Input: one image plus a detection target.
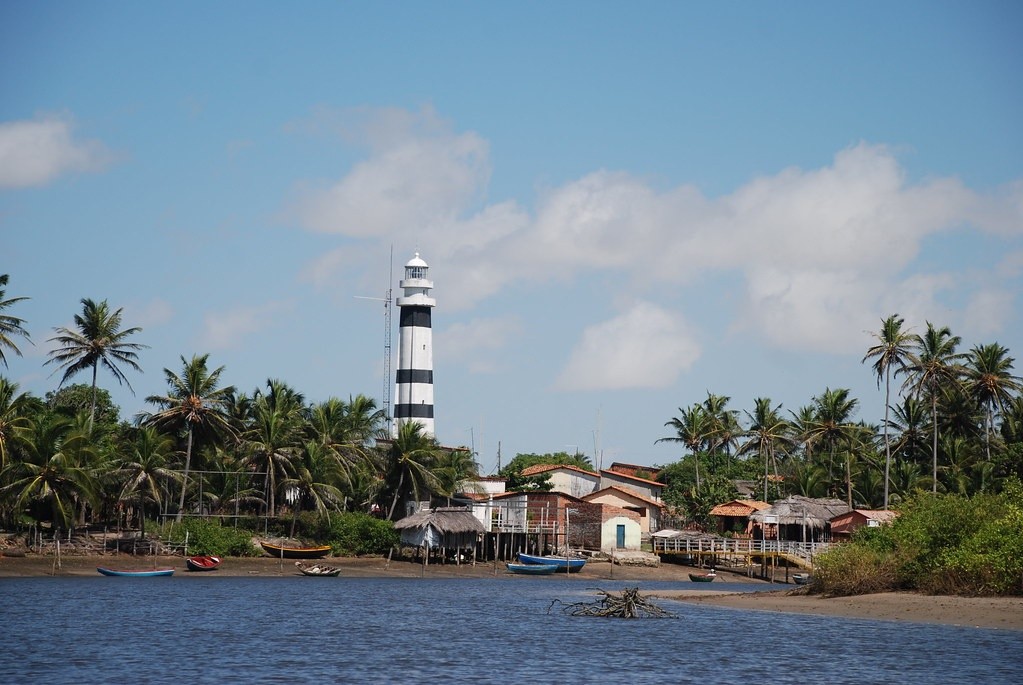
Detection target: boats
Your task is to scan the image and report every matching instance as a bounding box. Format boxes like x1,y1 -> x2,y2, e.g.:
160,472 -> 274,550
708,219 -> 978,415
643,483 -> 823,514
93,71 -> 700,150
504,561 -> 560,576
515,551 -> 587,573
793,573 -> 814,584
186,554 -> 222,571
295,561 -> 342,577
95,564 -> 177,577
687,572 -> 717,583
259,540 -> 334,559
